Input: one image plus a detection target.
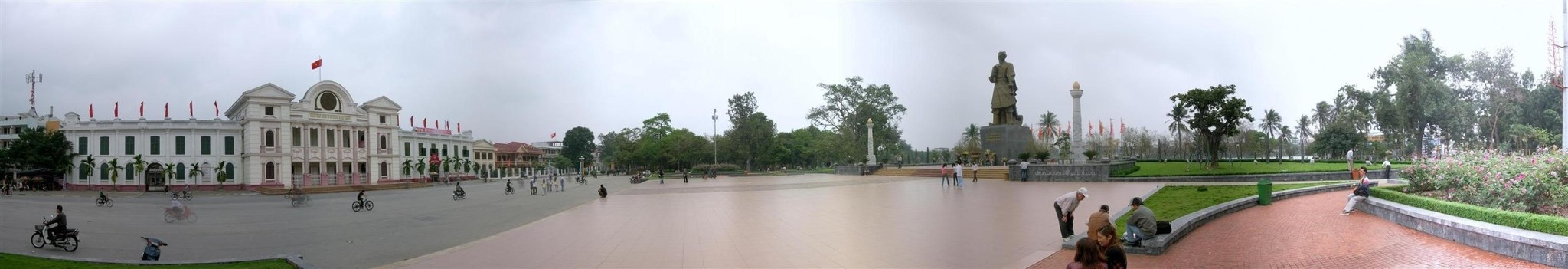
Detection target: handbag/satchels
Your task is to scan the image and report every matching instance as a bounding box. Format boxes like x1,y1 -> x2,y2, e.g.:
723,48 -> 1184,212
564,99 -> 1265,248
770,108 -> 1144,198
1155,220 -> 1172,234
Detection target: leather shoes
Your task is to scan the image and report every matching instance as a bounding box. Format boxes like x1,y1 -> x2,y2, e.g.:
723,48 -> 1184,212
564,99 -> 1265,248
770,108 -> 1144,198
1123,242 -> 1137,246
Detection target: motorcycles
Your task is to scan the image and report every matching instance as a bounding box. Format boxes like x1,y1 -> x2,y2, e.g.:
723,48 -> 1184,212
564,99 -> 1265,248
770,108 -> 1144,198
593,174 -> 598,178
290,195 -> 313,208
140,235 -> 167,261
533,176 -> 538,181
32,214 -> 81,252
629,170 -> 653,184
284,187 -> 303,199
164,204 -> 197,224
452,188 -> 467,201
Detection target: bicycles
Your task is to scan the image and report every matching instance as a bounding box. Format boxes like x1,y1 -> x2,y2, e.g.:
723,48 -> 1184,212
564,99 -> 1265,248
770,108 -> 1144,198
505,184 -> 515,195
580,178 -> 589,185
0,186 -> 13,197
171,191 -> 194,201
352,195 -> 374,212
96,194 -> 115,208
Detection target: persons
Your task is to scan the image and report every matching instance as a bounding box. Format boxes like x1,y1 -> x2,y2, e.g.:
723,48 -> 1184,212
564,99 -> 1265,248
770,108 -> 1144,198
3,174 -> 190,241
357,190 -> 368,209
1054,187 -> 1157,269
989,51 -> 1018,125
767,164 -> 801,172
290,184 -> 304,202
444,166 -> 716,198
898,154 -> 1031,190
1340,148 -> 1390,215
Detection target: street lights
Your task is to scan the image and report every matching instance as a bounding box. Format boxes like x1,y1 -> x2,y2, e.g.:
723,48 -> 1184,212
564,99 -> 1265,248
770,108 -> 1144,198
579,155 -> 585,177
1069,81 -> 1088,165
712,108 -> 719,166
631,159 -> 633,174
866,118 -> 875,165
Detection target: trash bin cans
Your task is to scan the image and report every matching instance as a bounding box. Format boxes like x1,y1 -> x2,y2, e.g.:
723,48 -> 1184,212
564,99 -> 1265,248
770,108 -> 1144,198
1352,168 -> 1359,180
1257,178 -> 1273,206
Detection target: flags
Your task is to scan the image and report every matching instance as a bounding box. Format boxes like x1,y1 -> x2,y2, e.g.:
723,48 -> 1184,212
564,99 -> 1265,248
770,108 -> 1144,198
1031,118 -> 1125,141
311,59 -> 322,69
89,101 -> 218,118
398,115 -> 460,132
551,132 -> 556,138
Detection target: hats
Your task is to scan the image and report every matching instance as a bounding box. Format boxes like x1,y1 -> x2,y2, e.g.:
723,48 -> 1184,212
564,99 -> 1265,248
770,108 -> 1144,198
1078,187 -> 1089,198
1130,197 -> 1142,206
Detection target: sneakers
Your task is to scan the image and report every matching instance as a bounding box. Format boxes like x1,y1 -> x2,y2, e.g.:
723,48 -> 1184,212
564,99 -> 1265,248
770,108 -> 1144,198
1069,234 -> 1075,238
1349,209 -> 1354,213
1339,209 -> 1349,215
1062,237 -> 1070,242
